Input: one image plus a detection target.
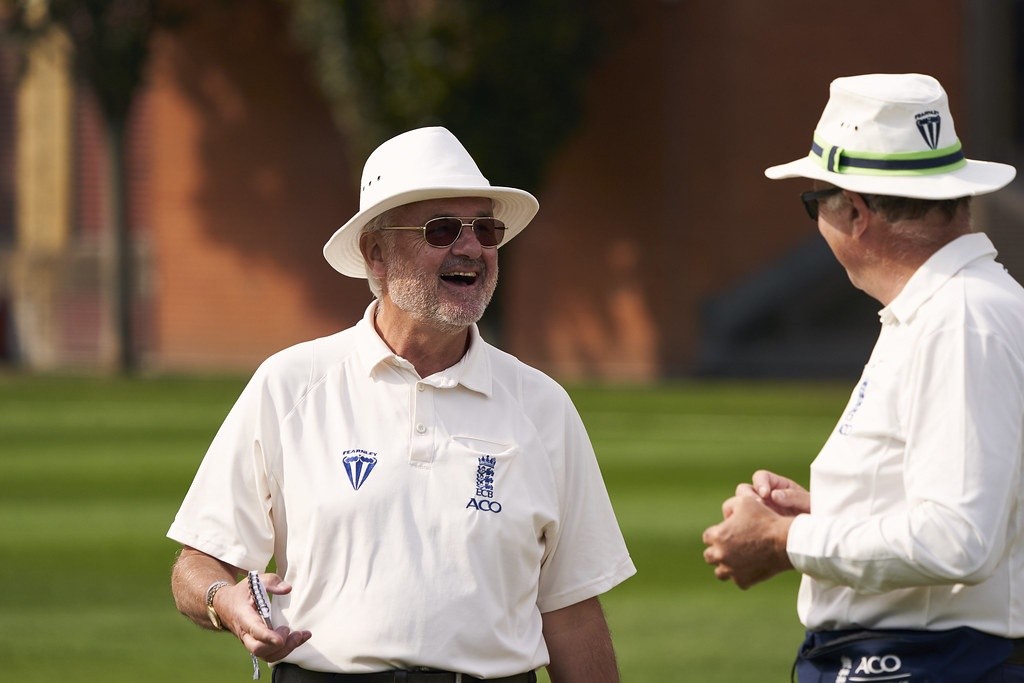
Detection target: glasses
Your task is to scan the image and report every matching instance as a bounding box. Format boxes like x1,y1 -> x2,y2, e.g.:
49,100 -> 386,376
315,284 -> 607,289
800,188 -> 842,221
379,217 -> 508,249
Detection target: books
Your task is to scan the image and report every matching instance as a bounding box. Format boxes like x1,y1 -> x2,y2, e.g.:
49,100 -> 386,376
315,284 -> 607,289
248,571 -> 274,630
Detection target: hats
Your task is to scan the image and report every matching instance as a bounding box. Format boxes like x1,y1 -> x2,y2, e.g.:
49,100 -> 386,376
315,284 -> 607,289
763,73 -> 1016,207
323,124 -> 541,280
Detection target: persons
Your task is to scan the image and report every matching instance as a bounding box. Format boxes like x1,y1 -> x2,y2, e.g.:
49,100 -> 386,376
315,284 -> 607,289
702,74 -> 1024,683
165,127 -> 638,683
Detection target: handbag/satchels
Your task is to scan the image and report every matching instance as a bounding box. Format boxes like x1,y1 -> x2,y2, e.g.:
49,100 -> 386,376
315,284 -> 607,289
790,622 -> 1017,683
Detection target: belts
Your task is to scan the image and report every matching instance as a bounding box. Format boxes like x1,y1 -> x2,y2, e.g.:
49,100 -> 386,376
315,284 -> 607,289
343,667 -> 537,683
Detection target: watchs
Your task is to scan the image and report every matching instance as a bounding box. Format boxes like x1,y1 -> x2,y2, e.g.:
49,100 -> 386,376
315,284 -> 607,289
206,581 -> 233,632
